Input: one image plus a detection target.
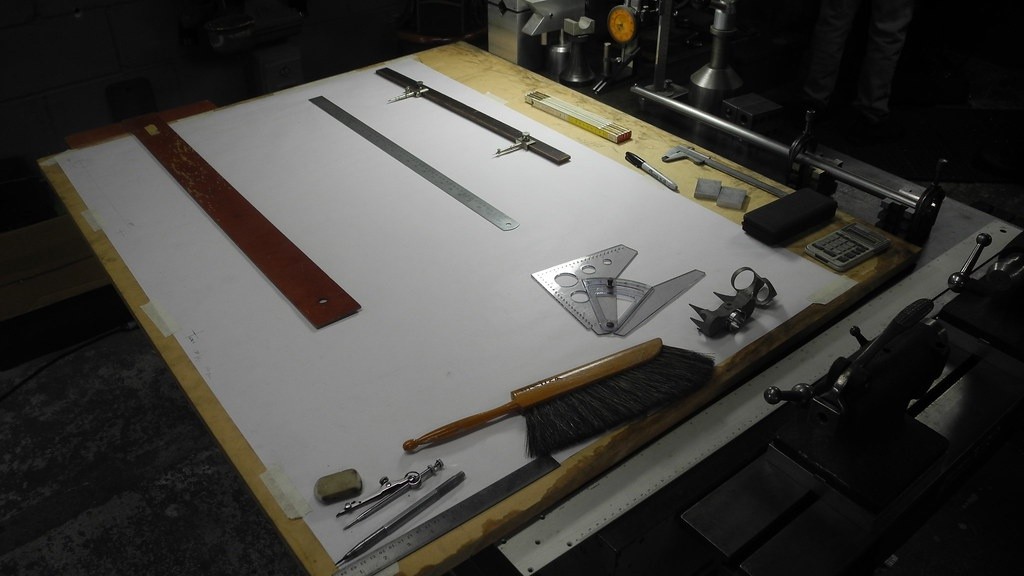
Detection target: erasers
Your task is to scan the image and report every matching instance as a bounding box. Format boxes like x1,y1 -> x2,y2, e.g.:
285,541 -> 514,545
313,469 -> 363,505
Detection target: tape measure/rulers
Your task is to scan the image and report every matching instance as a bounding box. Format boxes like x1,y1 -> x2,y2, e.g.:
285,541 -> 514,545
530,244 -> 707,336
308,96 -> 520,232
330,453 -> 561,576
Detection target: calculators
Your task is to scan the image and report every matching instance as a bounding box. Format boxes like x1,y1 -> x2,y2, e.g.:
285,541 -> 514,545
805,222 -> 892,272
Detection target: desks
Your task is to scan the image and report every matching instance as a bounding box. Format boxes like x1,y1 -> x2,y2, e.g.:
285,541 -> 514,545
35,34 -> 922,576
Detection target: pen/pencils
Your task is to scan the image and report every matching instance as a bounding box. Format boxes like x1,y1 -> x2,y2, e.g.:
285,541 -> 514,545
625,151 -> 678,191
